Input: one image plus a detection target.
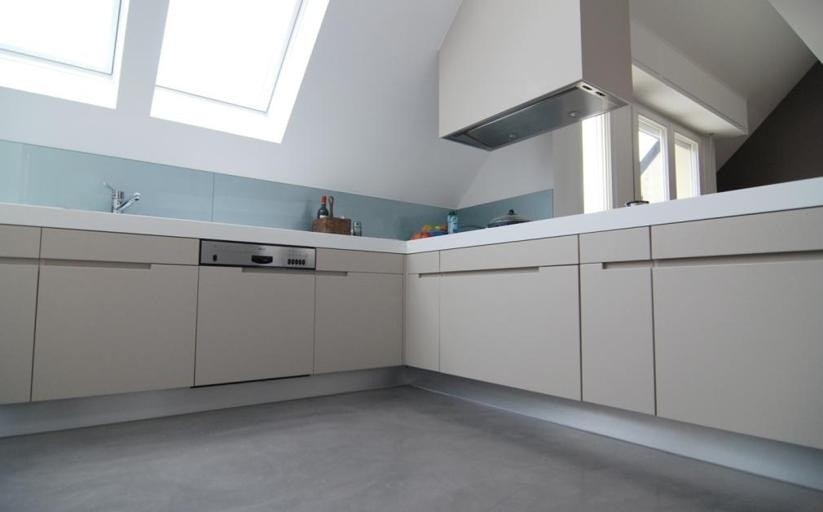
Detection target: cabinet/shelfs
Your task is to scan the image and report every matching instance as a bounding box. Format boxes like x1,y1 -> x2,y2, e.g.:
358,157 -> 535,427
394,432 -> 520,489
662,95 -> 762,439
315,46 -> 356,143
196,268 -> 314,390
651,205 -> 823,449
1,224 -> 200,408
402,249 -> 440,372
581,226 -> 656,416
315,248 -> 402,377
441,233 -> 580,402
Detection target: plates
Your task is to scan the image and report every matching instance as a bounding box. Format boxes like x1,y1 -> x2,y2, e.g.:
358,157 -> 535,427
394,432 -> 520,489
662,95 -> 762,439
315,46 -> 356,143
453,226 -> 481,231
426,231 -> 447,236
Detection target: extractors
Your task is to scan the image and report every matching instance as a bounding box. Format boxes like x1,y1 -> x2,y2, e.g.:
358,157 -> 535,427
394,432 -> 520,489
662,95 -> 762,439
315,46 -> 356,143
438,0 -> 633,152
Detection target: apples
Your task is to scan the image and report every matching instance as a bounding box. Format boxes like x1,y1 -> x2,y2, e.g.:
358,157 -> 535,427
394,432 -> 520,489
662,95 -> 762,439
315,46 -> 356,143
429,225 -> 447,231
410,233 -> 431,240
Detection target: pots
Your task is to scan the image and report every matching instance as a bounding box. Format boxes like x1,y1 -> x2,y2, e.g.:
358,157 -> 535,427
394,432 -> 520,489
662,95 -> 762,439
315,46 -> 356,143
488,208 -> 529,226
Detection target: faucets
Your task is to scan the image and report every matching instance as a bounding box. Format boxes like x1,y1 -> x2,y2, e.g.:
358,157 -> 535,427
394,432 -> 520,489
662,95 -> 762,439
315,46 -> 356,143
101,180 -> 141,212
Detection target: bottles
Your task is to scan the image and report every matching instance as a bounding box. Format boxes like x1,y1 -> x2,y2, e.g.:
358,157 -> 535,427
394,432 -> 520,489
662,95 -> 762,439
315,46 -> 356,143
317,192 -> 328,218
353,220 -> 362,236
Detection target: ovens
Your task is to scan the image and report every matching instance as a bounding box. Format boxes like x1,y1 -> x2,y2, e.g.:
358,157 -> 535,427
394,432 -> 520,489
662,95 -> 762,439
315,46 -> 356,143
197,237 -> 317,389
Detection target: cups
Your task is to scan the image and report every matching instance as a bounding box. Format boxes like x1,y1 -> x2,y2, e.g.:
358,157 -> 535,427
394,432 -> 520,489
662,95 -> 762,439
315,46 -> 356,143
446,209 -> 458,235
